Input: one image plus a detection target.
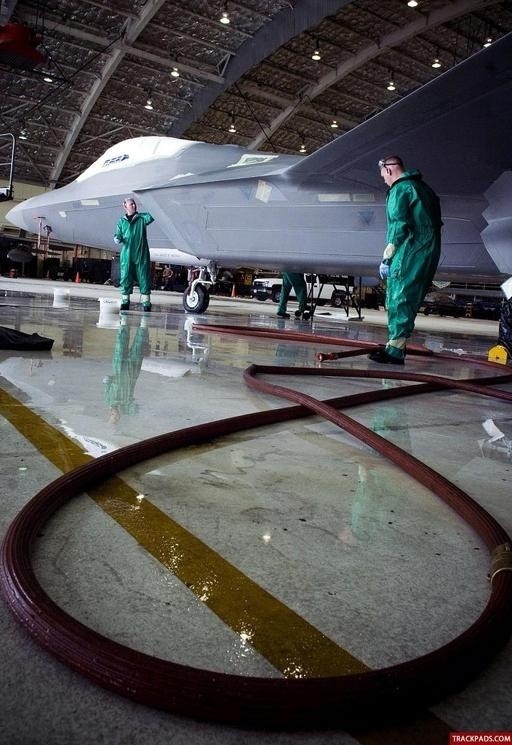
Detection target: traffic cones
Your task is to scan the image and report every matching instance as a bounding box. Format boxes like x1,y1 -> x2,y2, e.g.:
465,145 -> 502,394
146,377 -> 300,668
75,272 -> 81,284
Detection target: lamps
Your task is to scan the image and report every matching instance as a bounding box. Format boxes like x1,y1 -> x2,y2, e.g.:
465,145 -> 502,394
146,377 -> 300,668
387,0 -> 493,92
44,71 -> 53,84
18,130 -> 28,140
144,100 -> 154,110
220,0 -> 230,24
311,40 -> 321,61
299,138 -> 307,153
170,68 -> 180,78
229,116 -> 237,133
330,110 -> 339,129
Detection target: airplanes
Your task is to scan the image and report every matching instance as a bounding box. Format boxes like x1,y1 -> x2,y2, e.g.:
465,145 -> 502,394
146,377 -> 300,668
4,32 -> 511,313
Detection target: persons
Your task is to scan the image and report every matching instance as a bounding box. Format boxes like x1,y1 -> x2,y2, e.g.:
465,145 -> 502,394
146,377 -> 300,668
277,271 -> 315,318
161,264 -> 174,291
271,317 -> 310,368
221,268 -> 234,293
366,154 -> 444,365
334,364 -> 412,557
113,198 -> 157,311
101,310 -> 152,427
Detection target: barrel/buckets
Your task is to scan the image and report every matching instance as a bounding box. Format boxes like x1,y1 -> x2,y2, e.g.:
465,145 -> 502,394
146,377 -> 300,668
54,288 -> 71,309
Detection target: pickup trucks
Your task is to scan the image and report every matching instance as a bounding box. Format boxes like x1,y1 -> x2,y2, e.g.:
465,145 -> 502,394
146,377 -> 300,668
249,272 -> 357,308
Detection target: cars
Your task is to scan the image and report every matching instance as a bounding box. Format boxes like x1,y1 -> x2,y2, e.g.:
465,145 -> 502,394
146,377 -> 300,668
419,291 -> 468,318
471,300 -> 502,321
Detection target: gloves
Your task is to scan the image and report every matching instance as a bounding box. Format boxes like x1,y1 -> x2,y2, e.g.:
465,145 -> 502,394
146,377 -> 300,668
114,236 -> 121,246
379,262 -> 390,280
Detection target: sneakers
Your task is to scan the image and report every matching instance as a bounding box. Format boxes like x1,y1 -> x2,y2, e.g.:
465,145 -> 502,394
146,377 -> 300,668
370,350 -> 404,365
144,306 -> 153,313
277,312 -> 290,317
121,304 -> 129,310
